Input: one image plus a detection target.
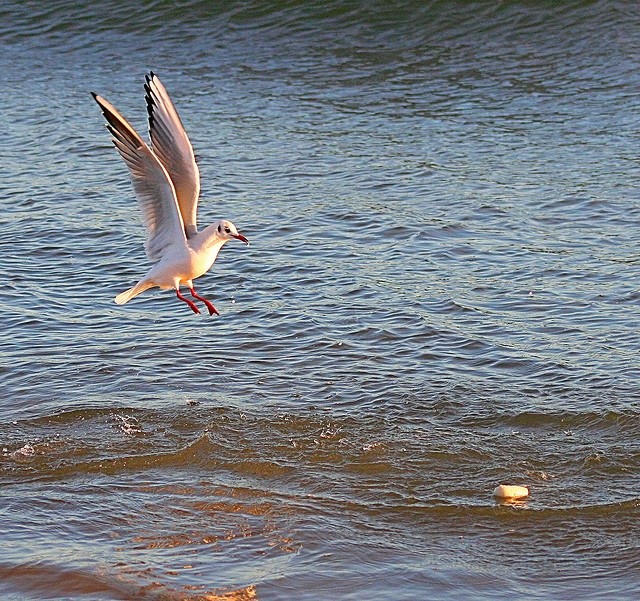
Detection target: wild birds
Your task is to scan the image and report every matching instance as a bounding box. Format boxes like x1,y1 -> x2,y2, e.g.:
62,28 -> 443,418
86,68 -> 252,319
